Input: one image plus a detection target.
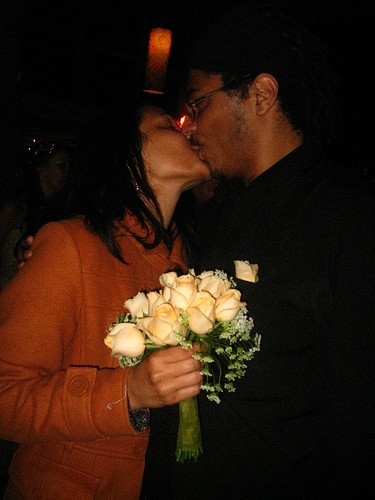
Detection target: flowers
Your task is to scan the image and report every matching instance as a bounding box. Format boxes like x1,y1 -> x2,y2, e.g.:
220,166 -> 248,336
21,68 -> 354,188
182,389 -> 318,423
105,259 -> 262,463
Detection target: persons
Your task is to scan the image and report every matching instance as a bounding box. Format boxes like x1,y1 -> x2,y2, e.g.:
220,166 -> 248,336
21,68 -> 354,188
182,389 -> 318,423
14,8 -> 375,500
0,103 -> 211,500
0,141 -> 75,288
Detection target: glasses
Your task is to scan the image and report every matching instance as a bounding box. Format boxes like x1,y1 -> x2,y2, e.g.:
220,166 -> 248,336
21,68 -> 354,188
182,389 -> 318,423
173,73 -> 258,128
